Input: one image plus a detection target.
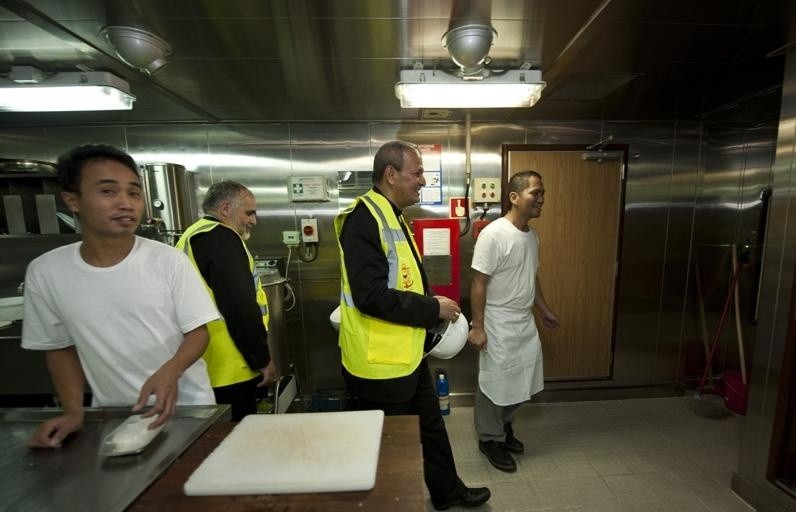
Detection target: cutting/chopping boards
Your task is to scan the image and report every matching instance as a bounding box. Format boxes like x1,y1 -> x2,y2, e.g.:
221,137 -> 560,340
180,410 -> 385,495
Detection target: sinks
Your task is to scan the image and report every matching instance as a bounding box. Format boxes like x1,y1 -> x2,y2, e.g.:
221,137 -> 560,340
0,297 -> 25,328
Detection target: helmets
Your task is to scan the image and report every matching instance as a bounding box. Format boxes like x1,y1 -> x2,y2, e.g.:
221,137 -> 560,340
421,312 -> 470,362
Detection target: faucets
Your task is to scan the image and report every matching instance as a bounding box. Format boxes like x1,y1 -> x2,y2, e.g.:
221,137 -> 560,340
17,281 -> 26,294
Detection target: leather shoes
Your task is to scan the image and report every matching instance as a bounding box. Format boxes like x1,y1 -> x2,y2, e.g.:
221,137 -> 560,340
479,438 -> 517,473
503,423 -> 525,452
434,488 -> 490,512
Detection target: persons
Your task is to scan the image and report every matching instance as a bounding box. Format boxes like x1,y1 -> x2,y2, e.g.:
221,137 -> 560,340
20,144 -> 220,449
174,181 -> 277,422
339,141 -> 490,510
468,171 -> 559,471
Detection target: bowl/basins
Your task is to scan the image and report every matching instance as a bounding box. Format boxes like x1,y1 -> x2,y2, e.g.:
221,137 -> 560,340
1,297 -> 21,324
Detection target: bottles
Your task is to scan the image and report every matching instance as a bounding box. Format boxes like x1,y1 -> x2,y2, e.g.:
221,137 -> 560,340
435,369 -> 451,416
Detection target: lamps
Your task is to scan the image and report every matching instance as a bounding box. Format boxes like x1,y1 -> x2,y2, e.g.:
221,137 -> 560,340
394,68 -> 547,110
1,70 -> 138,114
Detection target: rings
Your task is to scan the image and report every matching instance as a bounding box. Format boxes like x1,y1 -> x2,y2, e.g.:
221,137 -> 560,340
454,311 -> 460,318
